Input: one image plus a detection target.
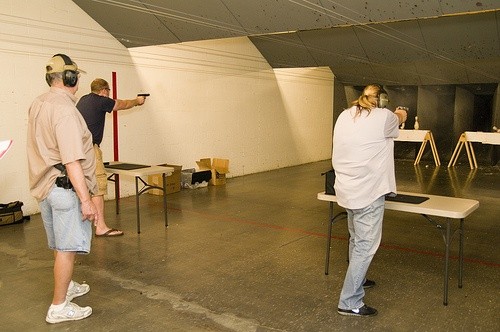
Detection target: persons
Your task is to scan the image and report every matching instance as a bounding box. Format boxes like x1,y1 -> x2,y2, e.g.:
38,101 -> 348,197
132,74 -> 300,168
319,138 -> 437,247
75,78 -> 146,236
26,54 -> 100,324
332,85 -> 407,317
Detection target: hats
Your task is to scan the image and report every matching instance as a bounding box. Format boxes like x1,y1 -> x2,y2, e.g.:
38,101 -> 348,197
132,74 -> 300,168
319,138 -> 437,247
45,53 -> 87,73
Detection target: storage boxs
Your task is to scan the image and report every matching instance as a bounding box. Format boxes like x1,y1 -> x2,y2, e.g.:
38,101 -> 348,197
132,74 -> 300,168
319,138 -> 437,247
147,164 -> 182,195
180,168 -> 211,190
196,157 -> 230,186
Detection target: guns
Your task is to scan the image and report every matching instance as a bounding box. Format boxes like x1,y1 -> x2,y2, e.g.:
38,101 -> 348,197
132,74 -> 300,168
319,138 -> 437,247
138,94 -> 150,96
397,106 -> 409,111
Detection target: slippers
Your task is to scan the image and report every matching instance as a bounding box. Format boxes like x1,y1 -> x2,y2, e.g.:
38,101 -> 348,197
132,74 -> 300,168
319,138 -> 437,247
95,228 -> 124,237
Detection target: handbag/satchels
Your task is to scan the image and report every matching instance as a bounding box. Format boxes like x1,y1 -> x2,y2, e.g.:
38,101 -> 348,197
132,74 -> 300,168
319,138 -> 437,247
0,200 -> 30,226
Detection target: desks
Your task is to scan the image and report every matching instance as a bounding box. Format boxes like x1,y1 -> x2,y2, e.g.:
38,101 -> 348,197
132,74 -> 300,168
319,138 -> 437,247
447,131 -> 500,170
317,191 -> 480,306
392,129 -> 440,167
100,161 -> 175,234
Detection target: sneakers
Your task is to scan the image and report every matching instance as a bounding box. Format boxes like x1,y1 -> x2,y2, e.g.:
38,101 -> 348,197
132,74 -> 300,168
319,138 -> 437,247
338,304 -> 378,317
362,279 -> 376,288
45,300 -> 93,324
66,280 -> 91,301
